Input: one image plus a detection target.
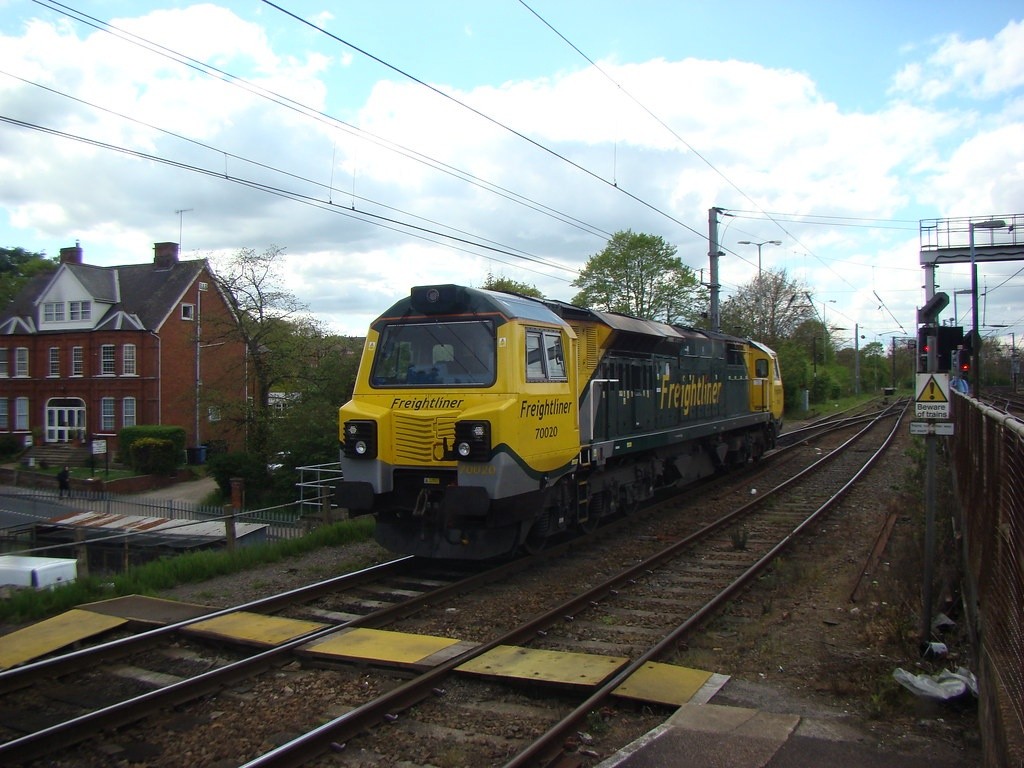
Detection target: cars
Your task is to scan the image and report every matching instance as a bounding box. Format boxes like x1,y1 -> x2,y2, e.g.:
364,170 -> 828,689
266,451 -> 292,477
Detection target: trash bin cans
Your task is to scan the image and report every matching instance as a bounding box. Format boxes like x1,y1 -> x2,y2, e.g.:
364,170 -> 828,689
193,446 -> 208,465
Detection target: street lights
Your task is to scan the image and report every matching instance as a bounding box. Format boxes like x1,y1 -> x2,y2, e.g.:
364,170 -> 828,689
736,238 -> 783,343
968,220 -> 1006,400
822,299 -> 837,365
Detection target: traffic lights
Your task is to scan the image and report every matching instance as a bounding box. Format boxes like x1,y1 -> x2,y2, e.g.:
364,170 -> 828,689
958,350 -> 970,373
917,326 -> 938,373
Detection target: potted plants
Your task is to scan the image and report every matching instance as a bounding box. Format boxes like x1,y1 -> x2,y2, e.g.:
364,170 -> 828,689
65,422 -> 81,447
33,425 -> 44,446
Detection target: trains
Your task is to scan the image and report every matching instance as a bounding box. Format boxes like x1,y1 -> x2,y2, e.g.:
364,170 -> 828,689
336,283 -> 784,566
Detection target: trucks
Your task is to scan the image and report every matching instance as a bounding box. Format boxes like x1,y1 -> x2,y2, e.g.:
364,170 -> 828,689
0,554 -> 78,590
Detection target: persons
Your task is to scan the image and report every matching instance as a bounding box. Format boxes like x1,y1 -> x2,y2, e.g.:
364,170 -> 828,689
57,465 -> 71,499
949,375 -> 968,396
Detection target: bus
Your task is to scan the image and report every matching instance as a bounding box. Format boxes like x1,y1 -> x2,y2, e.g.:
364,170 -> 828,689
267,393 -> 303,407
